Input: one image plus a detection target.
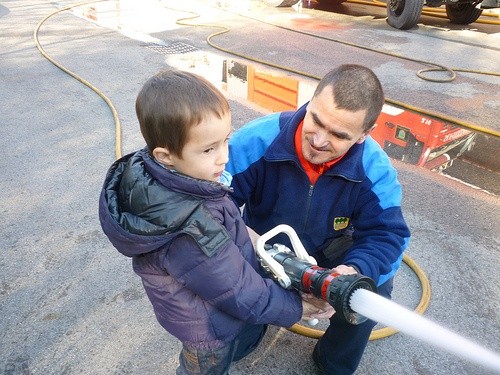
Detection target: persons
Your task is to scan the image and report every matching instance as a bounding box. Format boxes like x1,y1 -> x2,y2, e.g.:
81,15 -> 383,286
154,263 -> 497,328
214,63 -> 412,375
97,69 -> 328,375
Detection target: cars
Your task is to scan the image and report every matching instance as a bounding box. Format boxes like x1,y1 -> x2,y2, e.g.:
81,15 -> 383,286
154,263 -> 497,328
271,0 -> 500,31
